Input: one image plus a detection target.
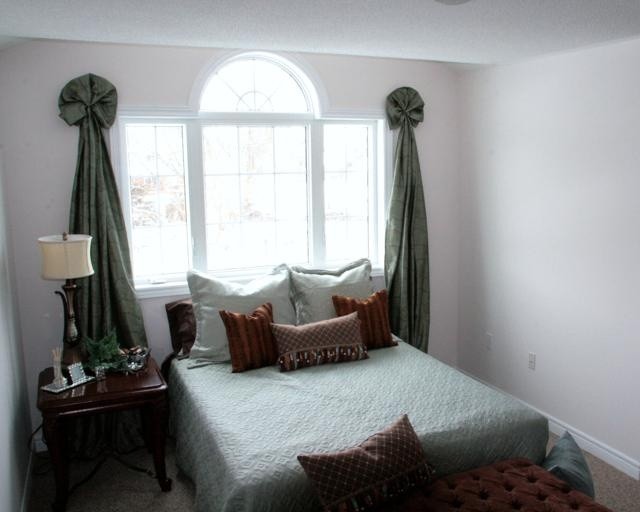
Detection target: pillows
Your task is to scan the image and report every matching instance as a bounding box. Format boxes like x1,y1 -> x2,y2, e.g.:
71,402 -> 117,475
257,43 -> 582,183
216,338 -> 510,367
297,415 -> 439,512
166,259 -> 399,372
542,430 -> 594,500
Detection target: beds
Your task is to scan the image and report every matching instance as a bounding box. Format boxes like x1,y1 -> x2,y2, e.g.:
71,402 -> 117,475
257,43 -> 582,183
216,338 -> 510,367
168,342 -> 549,512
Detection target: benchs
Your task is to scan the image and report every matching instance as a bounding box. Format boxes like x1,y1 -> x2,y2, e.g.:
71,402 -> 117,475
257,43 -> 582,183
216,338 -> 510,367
395,454 -> 612,512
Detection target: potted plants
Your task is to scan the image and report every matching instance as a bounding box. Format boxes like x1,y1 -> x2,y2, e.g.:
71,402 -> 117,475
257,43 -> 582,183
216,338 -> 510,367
82,328 -> 128,372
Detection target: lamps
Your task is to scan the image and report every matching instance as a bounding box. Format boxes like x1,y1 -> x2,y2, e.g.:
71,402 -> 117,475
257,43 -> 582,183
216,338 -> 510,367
38,233 -> 97,369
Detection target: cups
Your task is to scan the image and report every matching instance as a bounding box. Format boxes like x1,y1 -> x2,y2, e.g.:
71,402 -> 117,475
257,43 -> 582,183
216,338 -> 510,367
127,347 -> 152,375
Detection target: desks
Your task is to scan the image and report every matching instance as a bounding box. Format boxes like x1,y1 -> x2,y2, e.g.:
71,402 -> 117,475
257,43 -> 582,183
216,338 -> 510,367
37,356 -> 172,512
